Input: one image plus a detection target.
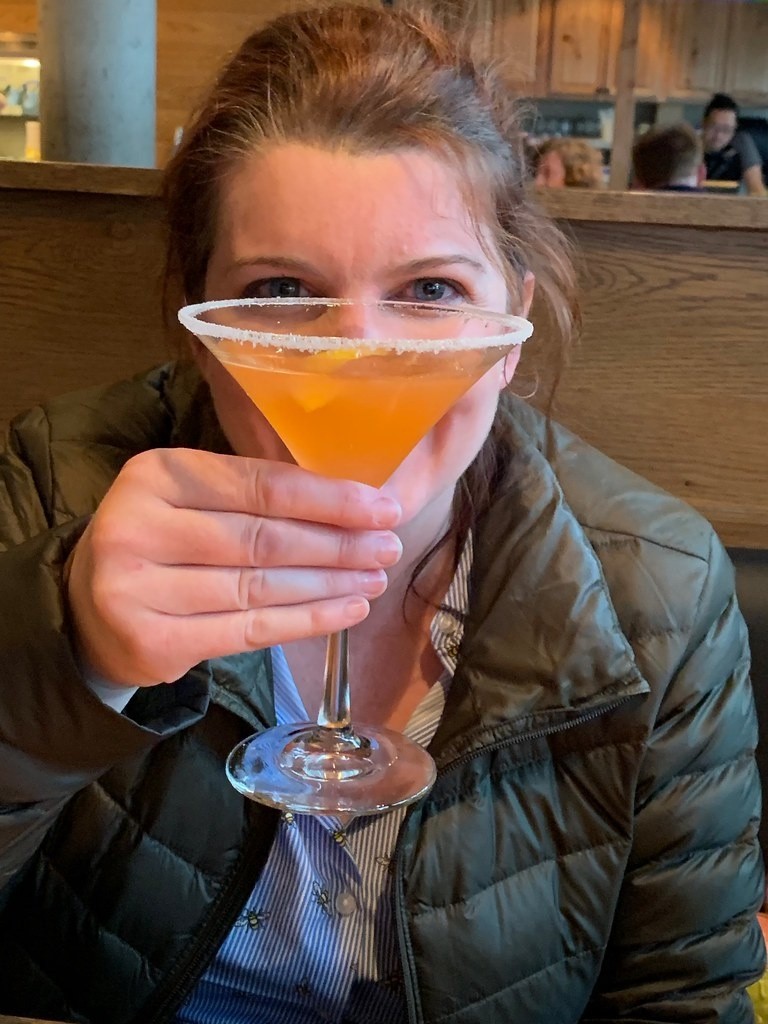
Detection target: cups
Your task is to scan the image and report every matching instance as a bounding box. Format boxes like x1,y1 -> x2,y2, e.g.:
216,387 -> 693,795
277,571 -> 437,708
0,28 -> 44,158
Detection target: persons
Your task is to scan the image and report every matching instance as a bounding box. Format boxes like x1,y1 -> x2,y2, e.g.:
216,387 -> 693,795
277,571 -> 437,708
692,93 -> 767,198
633,121 -> 713,194
530,137 -> 607,191
1,1 -> 768,1023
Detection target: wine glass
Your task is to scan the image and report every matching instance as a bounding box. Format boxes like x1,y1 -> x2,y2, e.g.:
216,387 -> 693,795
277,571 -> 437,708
170,294 -> 534,816
